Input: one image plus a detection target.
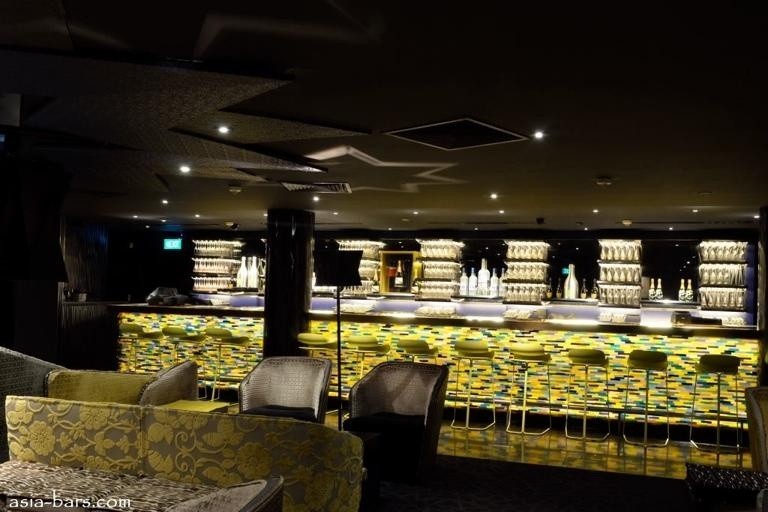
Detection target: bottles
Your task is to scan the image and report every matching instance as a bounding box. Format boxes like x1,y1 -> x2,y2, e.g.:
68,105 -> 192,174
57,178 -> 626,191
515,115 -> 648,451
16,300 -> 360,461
656,278 -> 663,300
686,279 -> 694,302
649,278 -> 655,303
556,277 -> 563,299
563,264 -> 579,300
677,277 -> 686,302
394,259 -> 403,288
590,278 -> 598,300
339,285 -> 367,299
579,278 -> 587,298
459,258 -> 504,299
236,255 -> 264,291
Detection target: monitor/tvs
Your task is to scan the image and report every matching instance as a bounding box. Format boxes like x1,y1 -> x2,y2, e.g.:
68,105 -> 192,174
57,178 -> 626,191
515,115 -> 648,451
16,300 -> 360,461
162,237 -> 182,252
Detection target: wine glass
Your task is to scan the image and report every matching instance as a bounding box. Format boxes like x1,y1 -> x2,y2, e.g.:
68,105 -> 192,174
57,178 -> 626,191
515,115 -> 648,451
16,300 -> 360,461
695,241 -> 748,312
503,239 -> 550,304
191,239 -> 241,292
416,237 -> 462,302
598,239 -> 643,308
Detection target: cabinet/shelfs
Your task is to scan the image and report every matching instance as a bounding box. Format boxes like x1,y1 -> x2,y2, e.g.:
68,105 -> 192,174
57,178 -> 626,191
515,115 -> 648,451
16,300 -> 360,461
502,238 -> 550,304
597,236 -> 642,308
187,234 -> 241,293
339,234 -> 464,301
697,240 -> 749,311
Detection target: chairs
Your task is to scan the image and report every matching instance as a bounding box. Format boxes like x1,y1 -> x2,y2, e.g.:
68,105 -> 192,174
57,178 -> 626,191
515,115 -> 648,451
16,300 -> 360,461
0,346 -> 451,510
115,318 -> 256,401
296,330 -> 746,475
